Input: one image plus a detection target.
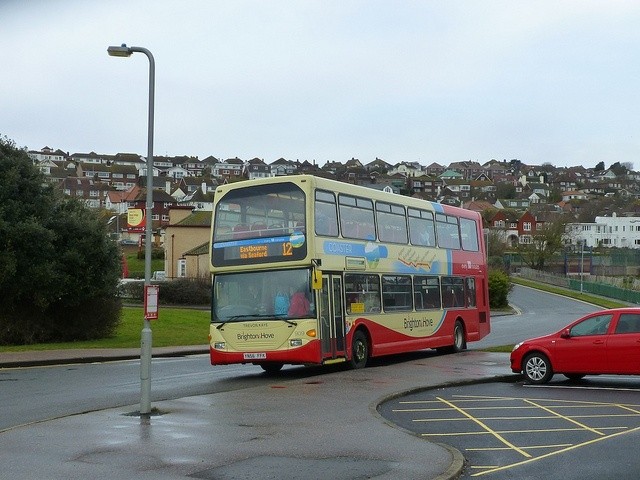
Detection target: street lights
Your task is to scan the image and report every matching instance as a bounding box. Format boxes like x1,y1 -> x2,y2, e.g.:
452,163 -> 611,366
106,43 -> 155,413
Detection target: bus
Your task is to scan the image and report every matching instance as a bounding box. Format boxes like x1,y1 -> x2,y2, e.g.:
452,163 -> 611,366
208,174 -> 491,369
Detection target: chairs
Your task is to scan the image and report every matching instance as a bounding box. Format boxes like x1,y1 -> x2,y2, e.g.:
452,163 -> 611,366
268,224 -> 283,236
250,221 -> 267,238
232,223 -> 250,238
295,220 -> 405,243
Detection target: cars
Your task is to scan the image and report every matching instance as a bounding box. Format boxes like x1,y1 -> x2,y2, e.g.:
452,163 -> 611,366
510,307 -> 640,385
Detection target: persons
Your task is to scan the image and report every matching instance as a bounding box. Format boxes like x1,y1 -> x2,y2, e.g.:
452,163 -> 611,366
420,228 -> 431,247
245,283 -> 259,305
275,285 -> 289,315
289,285 -> 310,317
